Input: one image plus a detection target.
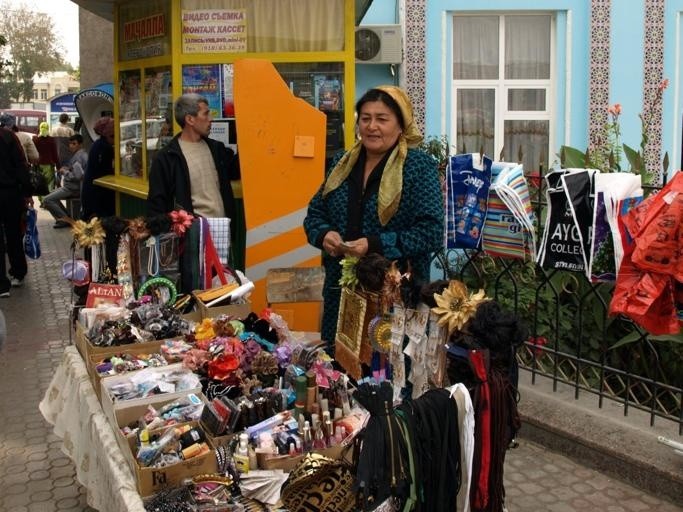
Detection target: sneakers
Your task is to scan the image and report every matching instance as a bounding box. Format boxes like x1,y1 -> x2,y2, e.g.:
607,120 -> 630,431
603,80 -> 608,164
0,271 -> 23,298
53,220 -> 72,228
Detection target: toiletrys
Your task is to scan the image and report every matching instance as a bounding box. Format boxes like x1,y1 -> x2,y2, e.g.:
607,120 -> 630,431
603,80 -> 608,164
234,373 -> 346,470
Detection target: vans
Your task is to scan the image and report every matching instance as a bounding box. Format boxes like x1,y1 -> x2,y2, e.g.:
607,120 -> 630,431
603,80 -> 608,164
0,108 -> 47,135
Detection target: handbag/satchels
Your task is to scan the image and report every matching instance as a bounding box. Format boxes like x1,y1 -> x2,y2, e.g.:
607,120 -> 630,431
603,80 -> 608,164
24,208 -> 42,260
444,152 -> 643,286
280,452 -> 359,512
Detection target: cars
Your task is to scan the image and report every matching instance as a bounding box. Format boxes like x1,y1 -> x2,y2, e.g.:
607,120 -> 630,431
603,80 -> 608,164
119,118 -> 161,175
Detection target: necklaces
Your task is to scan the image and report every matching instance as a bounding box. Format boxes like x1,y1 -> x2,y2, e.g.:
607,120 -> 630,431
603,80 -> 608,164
145,230 -> 177,278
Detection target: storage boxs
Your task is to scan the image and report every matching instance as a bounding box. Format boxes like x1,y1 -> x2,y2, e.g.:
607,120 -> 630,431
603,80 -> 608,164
200,421 -> 352,473
180,289 -> 250,322
75,319 -> 218,498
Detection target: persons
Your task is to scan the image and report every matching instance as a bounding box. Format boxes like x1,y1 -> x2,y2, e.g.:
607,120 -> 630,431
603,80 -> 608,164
36,121 -> 56,207
0,124 -> 34,297
43,134 -> 89,228
303,86 -> 445,379
52,114 -> 74,188
81,114 -> 114,262
12,124 -> 40,209
0,112 -> 16,253
145,93 -> 241,273
